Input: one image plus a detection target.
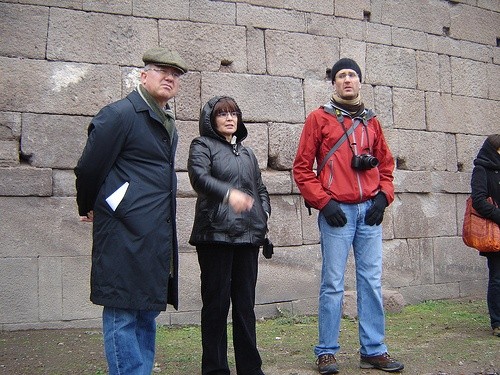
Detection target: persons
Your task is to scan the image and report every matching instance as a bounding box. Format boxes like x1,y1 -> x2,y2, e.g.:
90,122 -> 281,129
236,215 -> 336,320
74,48 -> 189,375
187,96 -> 271,375
471,134 -> 500,335
293,58 -> 404,374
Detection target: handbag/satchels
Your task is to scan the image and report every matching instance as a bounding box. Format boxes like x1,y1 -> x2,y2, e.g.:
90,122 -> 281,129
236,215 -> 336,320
462,194 -> 500,252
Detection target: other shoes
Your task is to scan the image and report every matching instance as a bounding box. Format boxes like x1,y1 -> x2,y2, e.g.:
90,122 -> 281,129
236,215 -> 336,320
494,325 -> 500,337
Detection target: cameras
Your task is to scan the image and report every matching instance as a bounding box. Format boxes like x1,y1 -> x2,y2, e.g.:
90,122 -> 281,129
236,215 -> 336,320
351,153 -> 379,170
263,237 -> 273,259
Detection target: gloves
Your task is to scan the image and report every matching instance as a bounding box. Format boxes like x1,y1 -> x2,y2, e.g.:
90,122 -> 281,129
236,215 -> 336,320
365,193 -> 388,226
321,199 -> 348,227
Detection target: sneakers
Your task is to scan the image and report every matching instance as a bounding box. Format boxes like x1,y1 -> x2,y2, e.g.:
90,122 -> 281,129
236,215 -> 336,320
360,352 -> 404,372
315,353 -> 339,374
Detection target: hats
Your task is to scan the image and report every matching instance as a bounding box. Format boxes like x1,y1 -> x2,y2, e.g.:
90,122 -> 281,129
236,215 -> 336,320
142,47 -> 188,74
332,58 -> 362,84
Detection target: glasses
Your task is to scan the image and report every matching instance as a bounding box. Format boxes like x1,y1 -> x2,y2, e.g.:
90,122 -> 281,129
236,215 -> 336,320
217,112 -> 237,119
147,68 -> 181,80
